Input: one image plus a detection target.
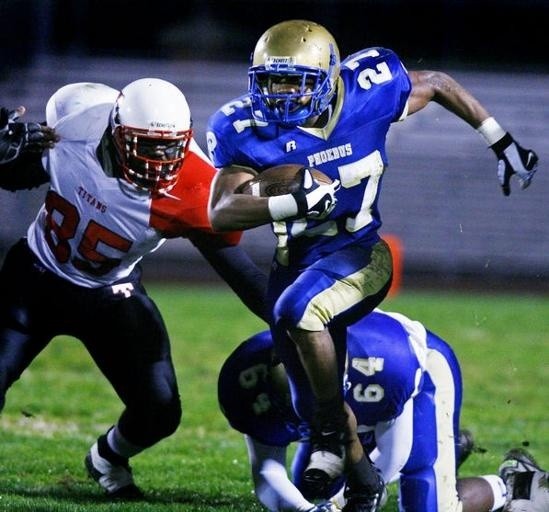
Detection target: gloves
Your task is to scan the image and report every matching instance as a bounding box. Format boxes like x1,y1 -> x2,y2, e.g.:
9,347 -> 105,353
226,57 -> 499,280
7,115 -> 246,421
307,502 -> 340,512
480,115 -> 537,196
0,107 -> 59,163
269,169 -> 342,221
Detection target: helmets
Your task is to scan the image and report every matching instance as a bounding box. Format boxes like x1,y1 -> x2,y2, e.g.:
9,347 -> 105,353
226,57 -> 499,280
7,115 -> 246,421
262,362 -> 304,425
110,78 -> 193,193
248,20 -> 341,123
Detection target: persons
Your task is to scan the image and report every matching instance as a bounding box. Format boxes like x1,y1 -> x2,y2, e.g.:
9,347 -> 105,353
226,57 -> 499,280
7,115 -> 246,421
2,77 -> 272,499
205,18 -> 539,512
219,305 -> 546,511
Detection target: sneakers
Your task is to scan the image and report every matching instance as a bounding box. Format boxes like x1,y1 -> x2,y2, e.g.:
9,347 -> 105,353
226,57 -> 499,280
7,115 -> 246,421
500,448 -> 542,478
339,475 -> 387,512
84,434 -> 141,500
302,430 -> 345,498
455,431 -> 472,470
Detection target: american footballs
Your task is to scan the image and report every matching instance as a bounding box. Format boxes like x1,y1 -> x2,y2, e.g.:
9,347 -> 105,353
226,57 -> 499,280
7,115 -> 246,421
240,164 -> 333,197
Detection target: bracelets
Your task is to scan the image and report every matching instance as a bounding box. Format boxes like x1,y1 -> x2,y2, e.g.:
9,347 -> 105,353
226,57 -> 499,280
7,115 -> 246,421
264,194 -> 299,220
476,115 -> 505,145
